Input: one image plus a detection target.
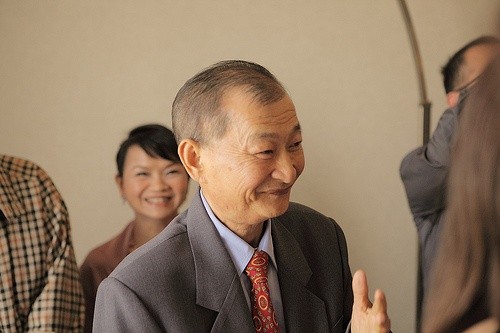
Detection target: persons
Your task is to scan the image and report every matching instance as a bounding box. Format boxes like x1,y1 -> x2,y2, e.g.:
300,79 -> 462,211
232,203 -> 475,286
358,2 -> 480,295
0,153 -> 88,333
421,51 -> 500,332
399,35 -> 500,308
91,59 -> 394,333
79,123 -> 189,333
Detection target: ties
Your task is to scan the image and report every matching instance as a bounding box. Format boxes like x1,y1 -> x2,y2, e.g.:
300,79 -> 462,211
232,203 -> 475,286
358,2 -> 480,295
244,250 -> 282,333
